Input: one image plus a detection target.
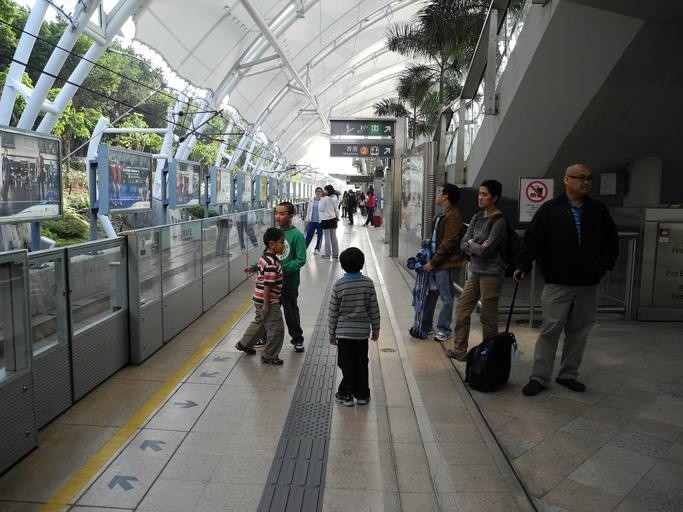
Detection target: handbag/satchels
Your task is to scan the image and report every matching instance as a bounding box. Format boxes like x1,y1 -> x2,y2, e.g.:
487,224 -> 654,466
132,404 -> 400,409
464,332 -> 514,392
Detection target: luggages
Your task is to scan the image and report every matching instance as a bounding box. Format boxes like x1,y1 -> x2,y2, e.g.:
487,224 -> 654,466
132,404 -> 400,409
373,210 -> 381,227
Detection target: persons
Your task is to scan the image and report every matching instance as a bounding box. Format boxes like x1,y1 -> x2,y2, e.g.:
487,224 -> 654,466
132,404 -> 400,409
235,200 -> 258,250
215,212 -> 233,257
242,201 -> 306,352
421,183 -> 466,340
235,227 -> 284,366
304,185 -> 379,258
328,247 -> 380,406
513,164 -> 619,396
0,148 -> 57,201
138,176 -> 151,202
446,179 -> 508,362
109,158 -> 128,206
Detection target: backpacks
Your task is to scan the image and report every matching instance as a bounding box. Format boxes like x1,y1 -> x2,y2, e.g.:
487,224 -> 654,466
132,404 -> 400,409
487,214 -> 519,265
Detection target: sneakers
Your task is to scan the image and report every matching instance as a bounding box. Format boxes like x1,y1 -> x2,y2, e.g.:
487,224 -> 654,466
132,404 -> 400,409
234,342 -> 255,355
555,378 -> 585,392
332,255 -> 337,258
260,355 -> 283,365
253,338 -> 265,348
522,379 -> 543,396
320,255 -> 329,259
294,343 -> 304,352
445,349 -> 466,362
357,396 -> 369,405
433,331 -> 447,341
313,249 -> 318,255
334,392 -> 353,407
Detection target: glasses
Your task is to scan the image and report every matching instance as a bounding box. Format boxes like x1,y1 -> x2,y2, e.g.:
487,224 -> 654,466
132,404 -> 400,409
566,174 -> 592,180
